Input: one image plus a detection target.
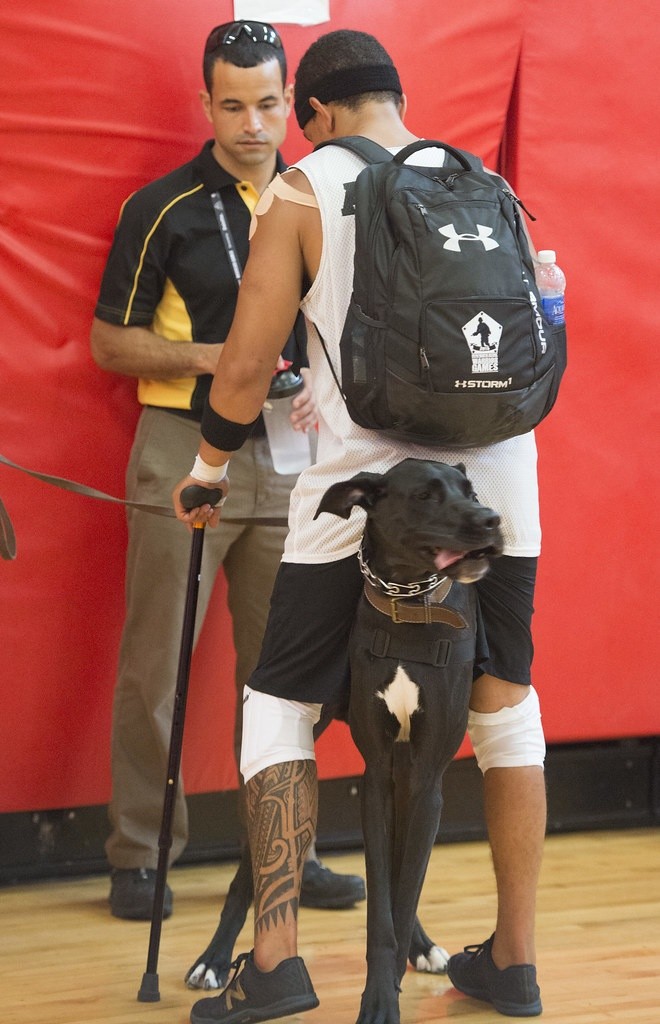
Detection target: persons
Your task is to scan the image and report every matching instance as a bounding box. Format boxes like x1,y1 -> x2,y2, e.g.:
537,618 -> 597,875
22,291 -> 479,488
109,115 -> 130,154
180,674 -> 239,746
88,23 -> 367,920
172,32 -> 546,1024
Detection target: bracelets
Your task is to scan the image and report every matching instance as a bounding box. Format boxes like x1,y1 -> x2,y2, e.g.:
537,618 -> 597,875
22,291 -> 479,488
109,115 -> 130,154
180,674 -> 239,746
187,454 -> 230,485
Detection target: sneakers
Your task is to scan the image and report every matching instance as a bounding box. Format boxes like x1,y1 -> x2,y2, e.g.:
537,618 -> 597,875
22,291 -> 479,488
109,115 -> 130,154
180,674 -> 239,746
447,932 -> 542,1016
108,867 -> 172,919
298,856 -> 366,907
190,947 -> 320,1024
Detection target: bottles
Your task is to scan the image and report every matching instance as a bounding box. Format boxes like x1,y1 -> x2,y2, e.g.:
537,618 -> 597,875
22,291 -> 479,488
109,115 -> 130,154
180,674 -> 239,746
534,249 -> 566,325
262,359 -> 312,473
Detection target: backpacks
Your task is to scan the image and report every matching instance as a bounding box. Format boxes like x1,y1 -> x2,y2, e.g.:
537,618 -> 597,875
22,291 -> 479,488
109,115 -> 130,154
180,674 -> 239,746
313,136 -> 567,450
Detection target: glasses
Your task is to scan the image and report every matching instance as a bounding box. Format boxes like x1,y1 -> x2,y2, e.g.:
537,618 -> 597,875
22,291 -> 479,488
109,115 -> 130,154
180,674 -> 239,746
205,21 -> 283,53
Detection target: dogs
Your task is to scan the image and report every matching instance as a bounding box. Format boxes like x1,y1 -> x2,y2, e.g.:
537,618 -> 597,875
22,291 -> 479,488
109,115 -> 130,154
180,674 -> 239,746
182,458 -> 504,1024
